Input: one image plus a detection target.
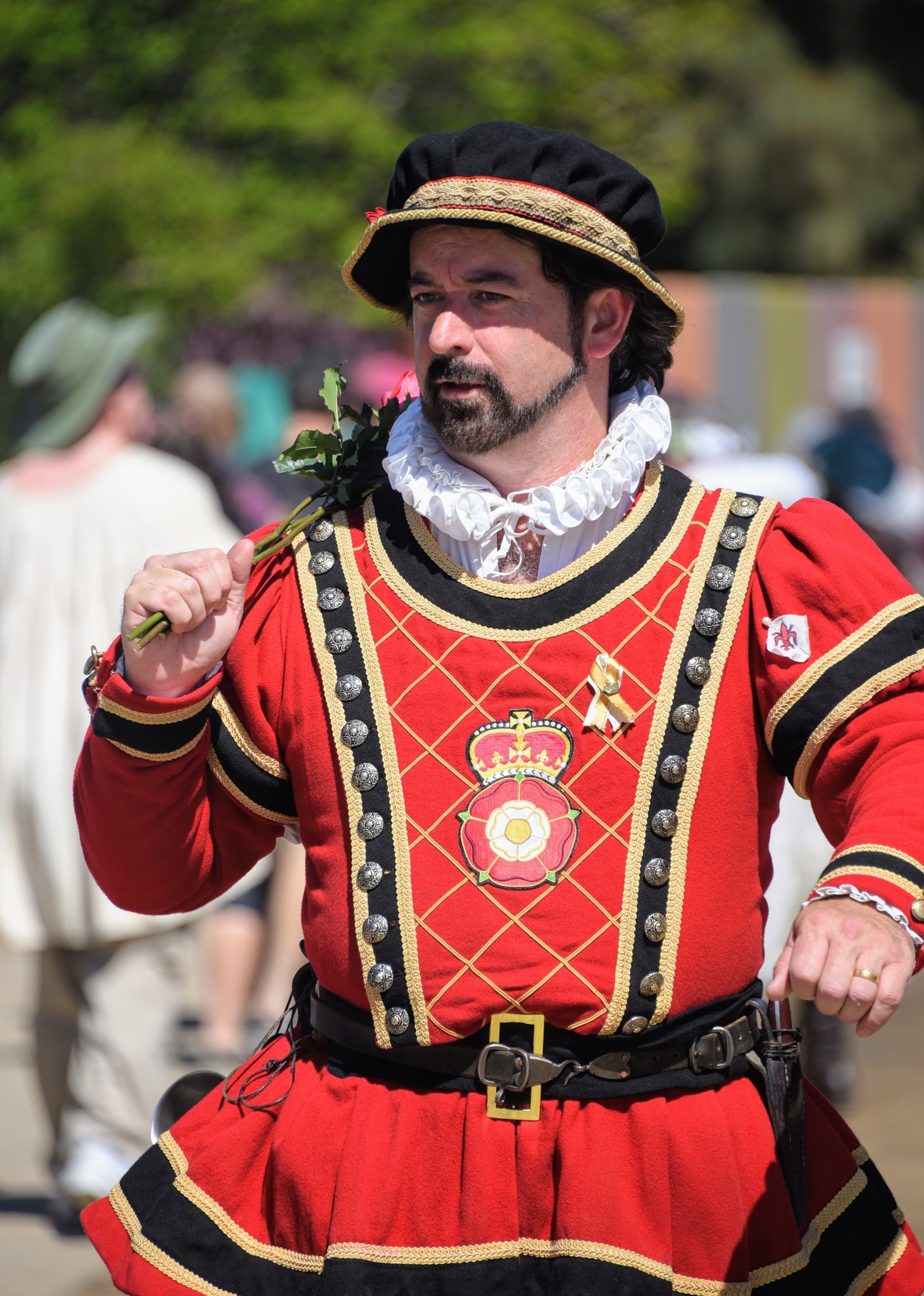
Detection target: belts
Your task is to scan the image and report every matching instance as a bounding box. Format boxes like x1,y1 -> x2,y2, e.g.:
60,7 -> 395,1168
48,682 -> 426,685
308,993 -> 760,1101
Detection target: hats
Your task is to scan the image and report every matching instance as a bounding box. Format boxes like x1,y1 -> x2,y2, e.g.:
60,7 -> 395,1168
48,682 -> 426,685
341,120 -> 684,345
8,295 -> 161,453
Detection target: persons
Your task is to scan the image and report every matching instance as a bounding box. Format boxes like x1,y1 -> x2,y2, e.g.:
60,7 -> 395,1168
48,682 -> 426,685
0,120 -> 924,1296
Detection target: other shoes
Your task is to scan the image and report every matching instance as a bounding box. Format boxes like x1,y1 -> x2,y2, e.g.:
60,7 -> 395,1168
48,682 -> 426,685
46,1193 -> 90,1236
0,1198 -> 46,1212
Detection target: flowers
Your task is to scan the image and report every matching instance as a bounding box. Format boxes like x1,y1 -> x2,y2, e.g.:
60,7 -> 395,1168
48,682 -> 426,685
125,360 -> 418,650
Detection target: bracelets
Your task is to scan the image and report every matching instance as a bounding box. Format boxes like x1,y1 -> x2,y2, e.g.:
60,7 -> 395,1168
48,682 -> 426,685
853,968 -> 879,985
